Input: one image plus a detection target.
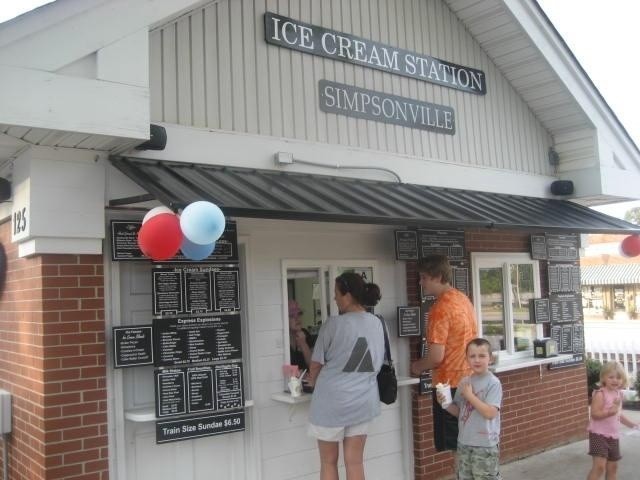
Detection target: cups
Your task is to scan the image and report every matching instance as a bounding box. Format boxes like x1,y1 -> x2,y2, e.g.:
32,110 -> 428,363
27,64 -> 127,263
288,380 -> 303,397
436,384 -> 453,404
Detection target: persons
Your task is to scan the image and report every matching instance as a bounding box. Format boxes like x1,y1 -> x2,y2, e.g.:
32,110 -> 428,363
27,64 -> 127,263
436,338 -> 504,480
302,272 -> 390,480
586,359 -> 640,480
411,254 -> 479,480
288,299 -> 318,373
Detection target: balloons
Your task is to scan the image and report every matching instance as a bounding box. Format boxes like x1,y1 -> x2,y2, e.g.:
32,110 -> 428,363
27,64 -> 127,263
180,201 -> 225,245
179,233 -> 215,261
142,205 -> 181,226
138,213 -> 183,260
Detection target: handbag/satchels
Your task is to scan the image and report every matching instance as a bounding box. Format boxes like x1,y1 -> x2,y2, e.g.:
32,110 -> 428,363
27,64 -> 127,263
377,364 -> 397,404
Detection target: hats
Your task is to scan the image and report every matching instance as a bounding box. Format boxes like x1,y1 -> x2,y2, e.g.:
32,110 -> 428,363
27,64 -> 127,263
288,300 -> 302,317
